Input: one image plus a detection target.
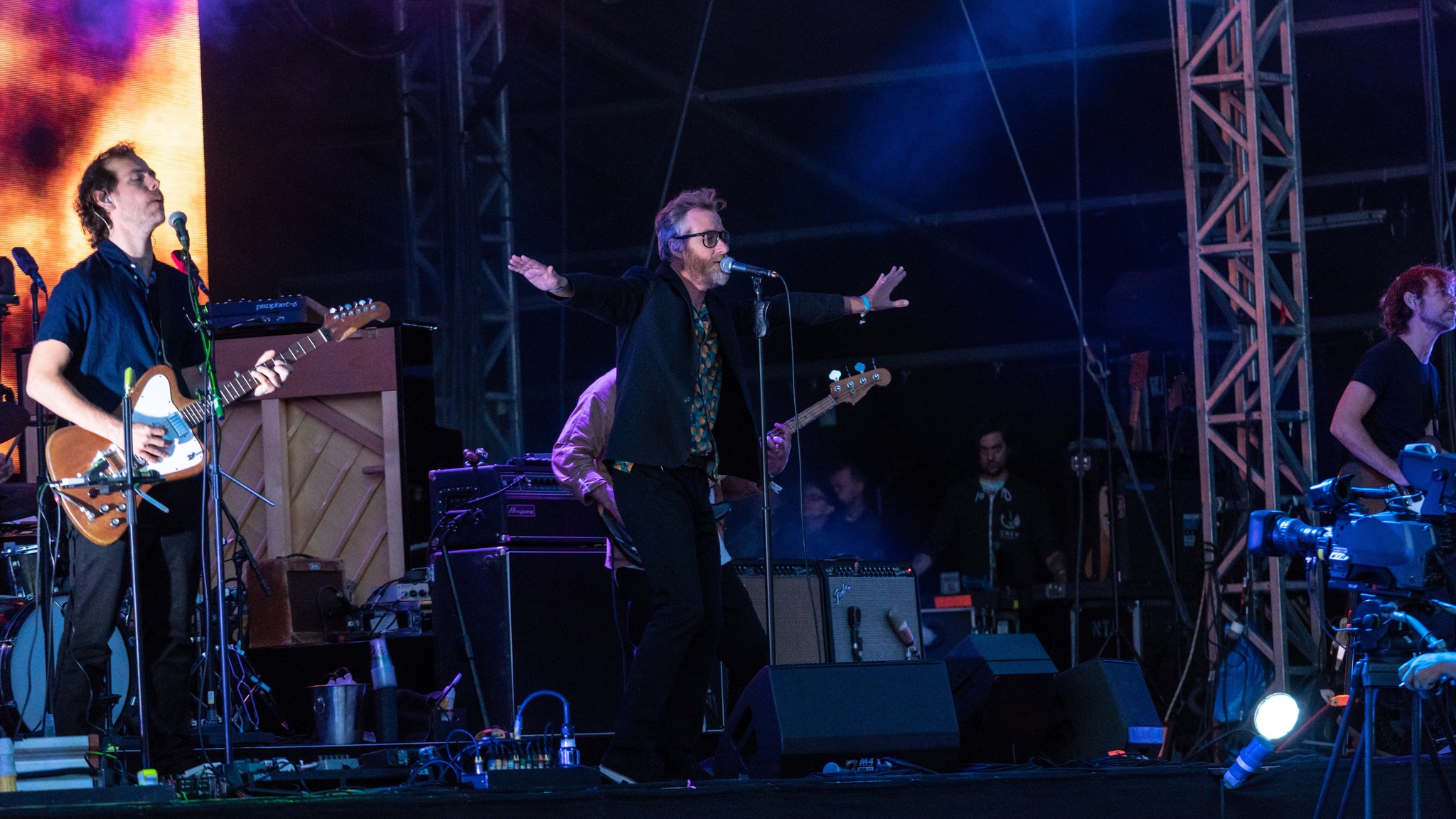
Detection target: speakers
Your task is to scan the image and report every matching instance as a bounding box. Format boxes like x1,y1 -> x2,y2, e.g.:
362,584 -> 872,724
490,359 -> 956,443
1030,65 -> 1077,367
941,632 -> 1061,763
1051,658 -> 1166,762
888,606 -> 922,657
243,552 -> 349,647
429,545 -> 628,738
717,658 -> 964,776
734,572 -> 925,662
848,606 -> 862,656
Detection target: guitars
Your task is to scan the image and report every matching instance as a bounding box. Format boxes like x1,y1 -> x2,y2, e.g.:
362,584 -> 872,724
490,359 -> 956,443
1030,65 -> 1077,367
43,295 -> 395,546
596,358 -> 892,568
1339,434 -> 1442,519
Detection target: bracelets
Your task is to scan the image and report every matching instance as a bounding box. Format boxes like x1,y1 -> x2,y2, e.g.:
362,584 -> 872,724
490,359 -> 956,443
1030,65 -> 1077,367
859,295 -> 871,317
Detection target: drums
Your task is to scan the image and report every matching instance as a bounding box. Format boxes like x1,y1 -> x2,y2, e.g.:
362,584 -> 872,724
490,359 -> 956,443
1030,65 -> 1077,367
1,543 -> 69,599
0,591 -> 139,737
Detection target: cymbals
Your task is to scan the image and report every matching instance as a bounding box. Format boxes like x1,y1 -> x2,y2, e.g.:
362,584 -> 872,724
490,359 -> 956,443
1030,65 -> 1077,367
0,401 -> 31,445
0,481 -> 40,524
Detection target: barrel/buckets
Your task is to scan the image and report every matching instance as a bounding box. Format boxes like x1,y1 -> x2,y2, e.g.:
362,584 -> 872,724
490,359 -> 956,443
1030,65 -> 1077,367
307,684 -> 367,745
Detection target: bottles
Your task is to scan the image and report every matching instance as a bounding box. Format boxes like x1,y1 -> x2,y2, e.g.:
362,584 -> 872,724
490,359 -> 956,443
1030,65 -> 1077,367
440,685 -> 456,721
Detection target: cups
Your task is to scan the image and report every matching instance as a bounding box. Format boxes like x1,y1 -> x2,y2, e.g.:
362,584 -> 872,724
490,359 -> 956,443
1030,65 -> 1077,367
369,638 -> 397,691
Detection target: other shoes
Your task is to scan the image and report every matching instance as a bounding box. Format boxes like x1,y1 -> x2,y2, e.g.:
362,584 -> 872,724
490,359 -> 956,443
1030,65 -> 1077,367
663,760 -> 714,781
600,730 -> 665,784
1337,703 -> 1406,757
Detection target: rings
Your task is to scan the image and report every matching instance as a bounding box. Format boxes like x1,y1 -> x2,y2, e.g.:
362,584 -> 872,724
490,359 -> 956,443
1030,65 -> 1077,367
773,448 -> 779,454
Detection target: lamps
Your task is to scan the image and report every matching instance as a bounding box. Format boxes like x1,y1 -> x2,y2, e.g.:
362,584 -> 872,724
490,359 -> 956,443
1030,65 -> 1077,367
1253,689 -> 1306,747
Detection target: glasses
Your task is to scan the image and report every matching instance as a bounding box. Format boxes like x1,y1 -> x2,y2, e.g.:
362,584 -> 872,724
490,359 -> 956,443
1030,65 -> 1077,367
674,230 -> 731,248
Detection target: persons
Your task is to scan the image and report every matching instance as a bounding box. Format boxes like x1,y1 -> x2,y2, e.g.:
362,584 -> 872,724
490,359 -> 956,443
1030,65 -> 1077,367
733,462 -> 906,562
1330,265 -> 1456,514
22,146 -> 293,780
506,187 -> 911,792
1398,652 -> 1456,690
552,365 -> 792,699
912,427 -> 1069,634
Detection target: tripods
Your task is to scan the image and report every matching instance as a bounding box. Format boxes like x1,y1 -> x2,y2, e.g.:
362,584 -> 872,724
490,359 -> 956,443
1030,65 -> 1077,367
1304,639 -> 1427,817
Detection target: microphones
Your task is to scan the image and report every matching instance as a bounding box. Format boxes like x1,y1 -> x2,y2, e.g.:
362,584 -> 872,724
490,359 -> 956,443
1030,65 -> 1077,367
169,211 -> 190,248
13,247 -> 47,292
719,256 -> 780,278
